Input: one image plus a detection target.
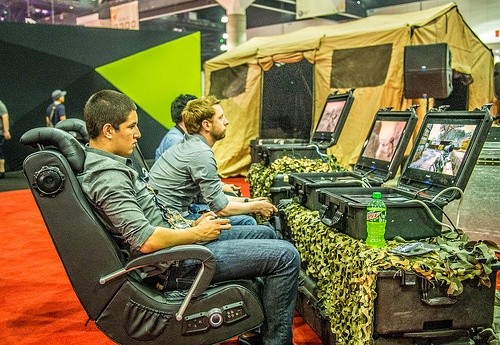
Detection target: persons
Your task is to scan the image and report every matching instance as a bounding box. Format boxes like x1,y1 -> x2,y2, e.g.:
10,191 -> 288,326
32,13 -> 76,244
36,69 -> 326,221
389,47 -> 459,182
46,90 -> 66,127
30,5 -> 41,19
0,100 -> 11,177
146,96 -> 278,240
155,93 -> 242,197
76,89 -> 301,345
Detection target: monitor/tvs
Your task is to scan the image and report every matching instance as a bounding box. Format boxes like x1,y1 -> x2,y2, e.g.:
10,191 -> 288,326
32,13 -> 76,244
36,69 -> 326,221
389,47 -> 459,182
397,108 -> 491,201
312,93 -> 354,143
354,109 -> 416,181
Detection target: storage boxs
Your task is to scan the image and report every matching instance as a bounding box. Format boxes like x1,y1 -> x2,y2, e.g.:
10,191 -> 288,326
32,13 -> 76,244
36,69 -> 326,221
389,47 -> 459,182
270,176 -> 293,208
293,247 -> 500,345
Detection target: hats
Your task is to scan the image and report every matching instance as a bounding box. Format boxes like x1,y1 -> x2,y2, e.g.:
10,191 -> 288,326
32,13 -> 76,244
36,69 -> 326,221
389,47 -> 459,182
51,90 -> 67,101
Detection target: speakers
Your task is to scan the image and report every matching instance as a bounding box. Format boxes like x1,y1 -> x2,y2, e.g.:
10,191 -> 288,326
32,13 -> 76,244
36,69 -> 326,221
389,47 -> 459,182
404,43 -> 453,100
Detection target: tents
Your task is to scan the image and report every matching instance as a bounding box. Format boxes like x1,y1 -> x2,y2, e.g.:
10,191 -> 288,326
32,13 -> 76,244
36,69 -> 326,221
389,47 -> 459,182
204,2 -> 496,178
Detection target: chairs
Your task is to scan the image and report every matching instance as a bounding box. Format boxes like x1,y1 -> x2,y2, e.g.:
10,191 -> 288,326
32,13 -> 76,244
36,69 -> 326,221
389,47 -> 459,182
55,118 -> 90,146
19,125 -> 266,344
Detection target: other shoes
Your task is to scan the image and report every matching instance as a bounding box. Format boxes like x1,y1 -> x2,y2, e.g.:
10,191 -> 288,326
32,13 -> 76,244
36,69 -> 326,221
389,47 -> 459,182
0,172 -> 7,178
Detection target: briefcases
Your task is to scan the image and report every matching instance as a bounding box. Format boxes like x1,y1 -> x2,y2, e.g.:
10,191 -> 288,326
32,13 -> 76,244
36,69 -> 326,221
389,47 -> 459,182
249,88 -> 355,165
289,104 -> 419,211
316,103 -> 494,240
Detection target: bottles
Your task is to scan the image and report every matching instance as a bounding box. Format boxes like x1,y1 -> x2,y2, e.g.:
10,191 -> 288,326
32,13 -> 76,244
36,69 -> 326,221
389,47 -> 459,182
366,191 -> 387,249
